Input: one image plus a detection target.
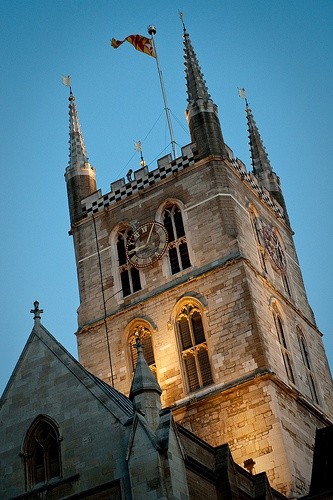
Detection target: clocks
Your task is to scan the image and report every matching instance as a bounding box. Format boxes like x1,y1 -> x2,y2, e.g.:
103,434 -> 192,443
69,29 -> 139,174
263,226 -> 288,273
125,221 -> 169,269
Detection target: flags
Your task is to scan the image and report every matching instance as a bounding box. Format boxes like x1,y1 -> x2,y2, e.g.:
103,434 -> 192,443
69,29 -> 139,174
132,141 -> 141,152
108,34 -> 156,58
237,88 -> 246,100
61,75 -> 70,87
